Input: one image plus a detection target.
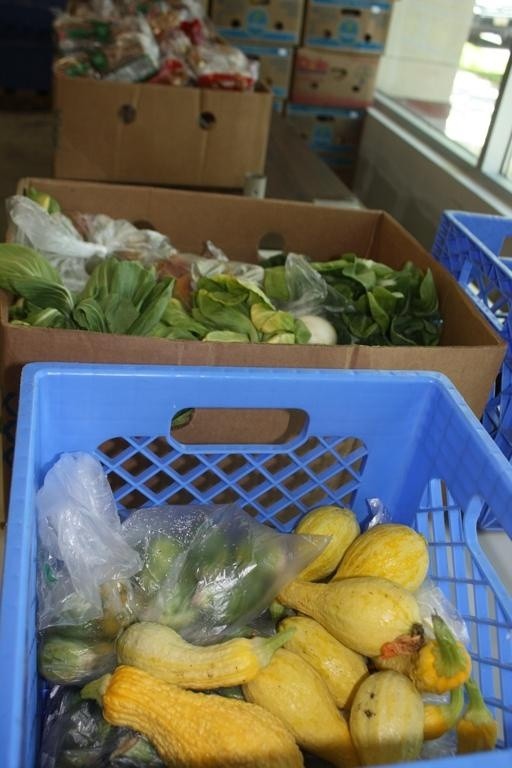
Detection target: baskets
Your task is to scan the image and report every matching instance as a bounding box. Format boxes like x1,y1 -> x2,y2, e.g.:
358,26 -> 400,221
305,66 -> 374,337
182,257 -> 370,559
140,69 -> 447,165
430,210 -> 511,532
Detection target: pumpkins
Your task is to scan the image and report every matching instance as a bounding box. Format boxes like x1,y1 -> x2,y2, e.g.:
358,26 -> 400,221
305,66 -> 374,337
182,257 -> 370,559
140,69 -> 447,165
37,502 -> 500,768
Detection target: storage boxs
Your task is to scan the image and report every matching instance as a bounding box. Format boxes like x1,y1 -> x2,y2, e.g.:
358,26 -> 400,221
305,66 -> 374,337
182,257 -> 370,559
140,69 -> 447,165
1,0 -> 510,530
